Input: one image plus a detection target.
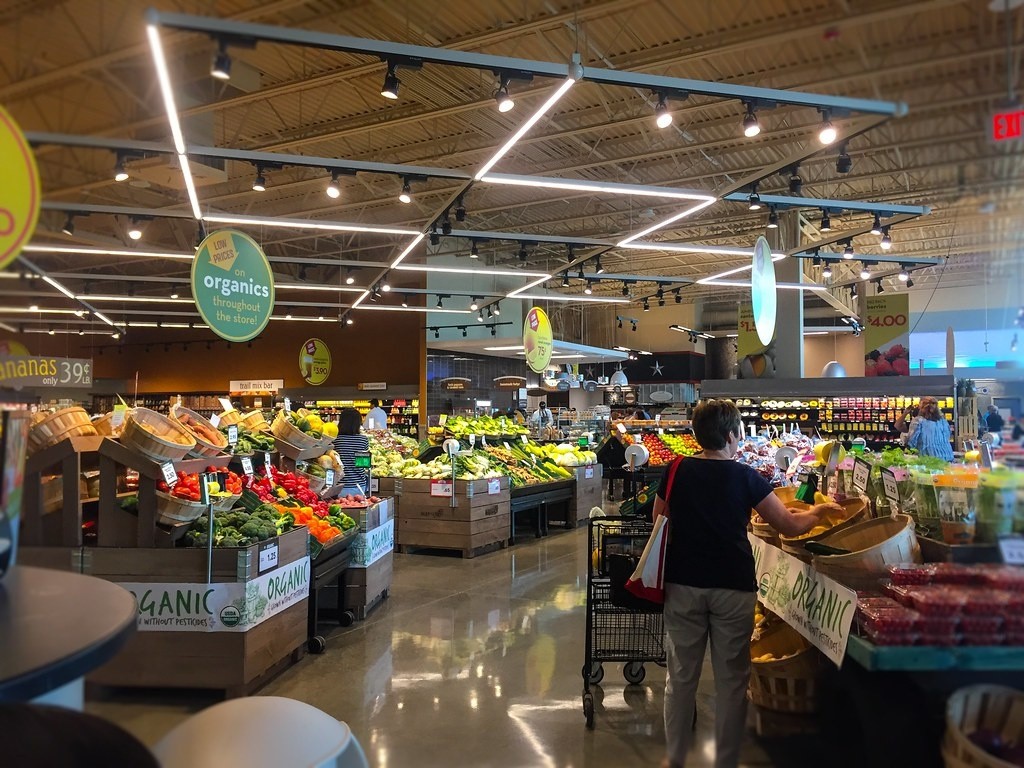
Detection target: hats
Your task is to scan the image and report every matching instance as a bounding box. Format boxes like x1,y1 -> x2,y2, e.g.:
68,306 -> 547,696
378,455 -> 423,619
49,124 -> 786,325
540,401 -> 546,409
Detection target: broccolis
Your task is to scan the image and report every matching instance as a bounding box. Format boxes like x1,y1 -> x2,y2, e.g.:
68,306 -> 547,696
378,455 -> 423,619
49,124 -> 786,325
185,503 -> 295,548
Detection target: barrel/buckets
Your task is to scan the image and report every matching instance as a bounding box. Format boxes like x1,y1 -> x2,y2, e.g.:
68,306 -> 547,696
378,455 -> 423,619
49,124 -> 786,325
0,405 -> 339,522
746,483 -> 1023,768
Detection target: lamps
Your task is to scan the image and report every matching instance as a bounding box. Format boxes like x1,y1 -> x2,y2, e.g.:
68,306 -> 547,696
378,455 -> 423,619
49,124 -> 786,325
28,5 -> 945,340
549,360 -> 630,387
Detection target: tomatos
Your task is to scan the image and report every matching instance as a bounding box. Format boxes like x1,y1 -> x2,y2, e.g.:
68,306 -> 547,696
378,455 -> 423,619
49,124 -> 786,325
853,561 -> 1024,647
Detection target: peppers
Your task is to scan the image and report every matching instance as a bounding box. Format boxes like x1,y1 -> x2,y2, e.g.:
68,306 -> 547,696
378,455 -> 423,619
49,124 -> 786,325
239,462 -> 355,542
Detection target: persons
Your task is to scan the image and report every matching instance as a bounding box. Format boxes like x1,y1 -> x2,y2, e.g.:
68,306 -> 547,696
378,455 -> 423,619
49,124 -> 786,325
531,400 -> 554,426
648,399 -> 849,768
893,403 -> 921,434
902,396 -> 956,462
492,405 -> 528,420
333,405 -> 369,497
976,403 -> 1024,447
608,404 -> 651,421
362,398 -> 387,429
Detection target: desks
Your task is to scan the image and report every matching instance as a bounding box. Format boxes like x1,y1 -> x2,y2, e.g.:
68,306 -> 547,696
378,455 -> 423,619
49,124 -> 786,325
1,564 -> 138,709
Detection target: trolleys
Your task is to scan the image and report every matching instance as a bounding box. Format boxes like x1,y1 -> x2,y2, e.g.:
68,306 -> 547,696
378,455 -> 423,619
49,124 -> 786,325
581,512 -> 669,724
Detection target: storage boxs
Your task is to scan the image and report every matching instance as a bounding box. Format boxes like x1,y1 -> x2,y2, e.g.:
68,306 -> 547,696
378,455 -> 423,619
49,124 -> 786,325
777,498 -> 877,569
748,625 -> 827,720
26,406 -> 98,457
210,409 -> 248,431
245,410 -> 268,432
120,406 -> 197,465
809,516 -> 927,593
271,408 -> 322,450
852,558 -> 1023,647
168,404 -> 229,461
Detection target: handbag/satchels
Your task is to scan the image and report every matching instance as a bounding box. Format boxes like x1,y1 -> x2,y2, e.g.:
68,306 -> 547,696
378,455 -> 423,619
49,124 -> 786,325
626,454 -> 685,603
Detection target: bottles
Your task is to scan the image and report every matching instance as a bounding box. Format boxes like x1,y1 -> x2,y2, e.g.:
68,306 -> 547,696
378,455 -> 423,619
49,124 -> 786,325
386,398 -> 420,440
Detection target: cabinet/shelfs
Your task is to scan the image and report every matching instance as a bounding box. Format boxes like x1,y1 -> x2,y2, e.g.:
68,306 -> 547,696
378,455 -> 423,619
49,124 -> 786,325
697,394 -> 956,455
299,400 -> 605,564
19,442 -> 396,701
590,418 -> 706,503
